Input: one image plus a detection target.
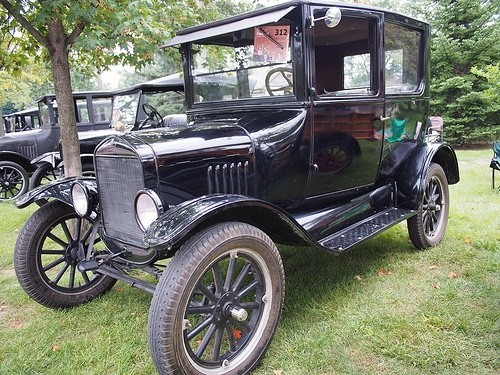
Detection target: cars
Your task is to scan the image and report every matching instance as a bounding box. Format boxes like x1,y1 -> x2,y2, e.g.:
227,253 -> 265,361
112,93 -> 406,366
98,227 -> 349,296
0,109 -> 39,133
15,0 -> 461,375
0,92 -> 112,201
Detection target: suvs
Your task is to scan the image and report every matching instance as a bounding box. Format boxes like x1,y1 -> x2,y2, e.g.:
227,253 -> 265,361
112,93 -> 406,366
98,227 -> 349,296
29,85 -> 235,209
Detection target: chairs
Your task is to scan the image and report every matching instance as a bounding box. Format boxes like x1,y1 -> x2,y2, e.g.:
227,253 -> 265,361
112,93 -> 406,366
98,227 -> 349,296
425,116 -> 444,143
489,142 -> 500,189
